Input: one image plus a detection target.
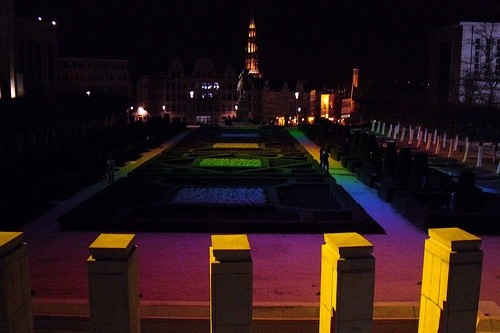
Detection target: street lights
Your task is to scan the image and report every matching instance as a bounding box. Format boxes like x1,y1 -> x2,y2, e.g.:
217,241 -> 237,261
295,91 -> 300,125
190,90 -> 195,125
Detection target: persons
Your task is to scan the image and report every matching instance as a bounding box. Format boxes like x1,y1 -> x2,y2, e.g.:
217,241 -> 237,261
319,141 -> 329,177
96,153 -> 116,183
